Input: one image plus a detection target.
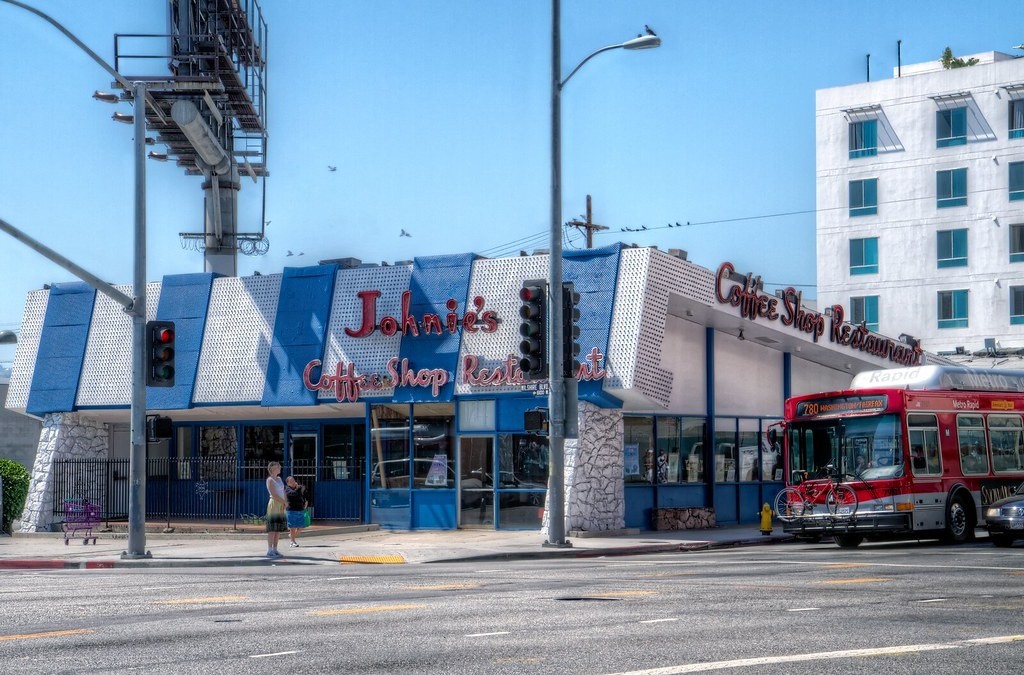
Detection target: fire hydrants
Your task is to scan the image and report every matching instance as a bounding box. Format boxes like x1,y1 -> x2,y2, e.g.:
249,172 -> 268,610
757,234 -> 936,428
756,503 -> 777,534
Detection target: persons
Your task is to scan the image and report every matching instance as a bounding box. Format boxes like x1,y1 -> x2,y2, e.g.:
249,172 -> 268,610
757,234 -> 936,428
656,448 -> 668,484
265,461 -> 289,558
284,477 -> 308,547
854,454 -> 872,478
914,445 -> 926,469
747,458 -> 764,481
771,454 -> 785,481
644,448 -> 655,484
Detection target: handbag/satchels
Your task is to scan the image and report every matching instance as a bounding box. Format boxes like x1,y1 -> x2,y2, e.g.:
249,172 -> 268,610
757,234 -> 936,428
303,509 -> 311,528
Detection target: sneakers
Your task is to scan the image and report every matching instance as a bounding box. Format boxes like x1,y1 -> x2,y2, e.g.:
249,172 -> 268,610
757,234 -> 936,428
290,542 -> 293,548
267,551 -> 283,558
293,542 -> 299,547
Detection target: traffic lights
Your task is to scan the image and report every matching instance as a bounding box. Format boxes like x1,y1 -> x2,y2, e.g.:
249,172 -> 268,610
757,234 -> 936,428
148,414 -> 173,440
146,321 -> 178,388
519,277 -> 546,379
562,279 -> 581,379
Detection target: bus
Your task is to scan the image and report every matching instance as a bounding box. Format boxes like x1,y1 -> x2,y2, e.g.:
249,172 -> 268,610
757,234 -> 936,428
765,356 -> 1024,544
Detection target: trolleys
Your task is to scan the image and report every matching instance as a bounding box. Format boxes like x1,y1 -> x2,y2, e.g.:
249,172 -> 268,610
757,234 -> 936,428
62,500 -> 103,545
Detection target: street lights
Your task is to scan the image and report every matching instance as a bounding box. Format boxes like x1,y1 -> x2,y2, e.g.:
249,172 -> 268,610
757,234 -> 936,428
545,33 -> 665,546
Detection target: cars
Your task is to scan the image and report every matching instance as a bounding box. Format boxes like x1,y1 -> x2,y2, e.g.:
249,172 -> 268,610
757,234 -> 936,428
986,480 -> 1024,549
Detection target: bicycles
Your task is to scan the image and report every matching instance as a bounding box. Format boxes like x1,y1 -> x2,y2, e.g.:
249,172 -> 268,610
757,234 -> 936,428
775,463 -> 860,523
